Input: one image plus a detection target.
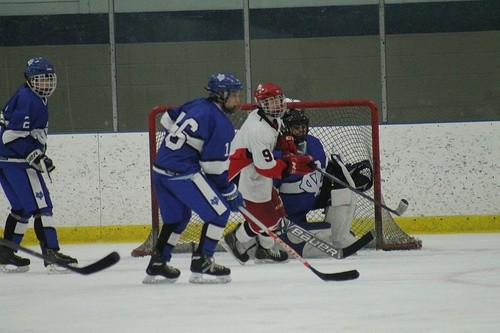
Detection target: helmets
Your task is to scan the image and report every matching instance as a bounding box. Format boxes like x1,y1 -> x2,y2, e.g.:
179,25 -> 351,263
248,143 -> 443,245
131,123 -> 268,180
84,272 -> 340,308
24,57 -> 57,98
205,73 -> 244,115
285,110 -> 308,142
255,83 -> 287,119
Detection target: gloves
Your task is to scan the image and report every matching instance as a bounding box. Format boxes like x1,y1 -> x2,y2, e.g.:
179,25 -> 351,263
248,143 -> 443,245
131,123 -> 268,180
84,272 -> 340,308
286,156 -> 317,175
281,132 -> 298,155
30,152 -> 56,174
226,192 -> 245,212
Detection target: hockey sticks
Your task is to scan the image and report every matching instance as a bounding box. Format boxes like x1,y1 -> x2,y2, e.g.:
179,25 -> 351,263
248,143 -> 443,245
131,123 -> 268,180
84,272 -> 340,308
40,160 -> 52,184
307,161 -> 409,216
283,216 -> 379,260
0,236 -> 121,276
237,205 -> 360,283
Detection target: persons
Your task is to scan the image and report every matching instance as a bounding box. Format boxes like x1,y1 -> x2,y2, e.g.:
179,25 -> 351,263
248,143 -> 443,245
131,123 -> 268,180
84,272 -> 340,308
271,108 -> 365,259
142,72 -> 246,284
0,59 -> 77,274
221,83 -> 317,263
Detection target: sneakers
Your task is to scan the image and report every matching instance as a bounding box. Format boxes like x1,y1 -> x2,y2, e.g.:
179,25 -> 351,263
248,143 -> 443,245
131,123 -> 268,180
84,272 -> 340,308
42,248 -> 78,273
255,238 -> 290,265
0,244 -> 31,273
220,222 -> 251,266
143,248 -> 180,285
190,241 -> 231,283
